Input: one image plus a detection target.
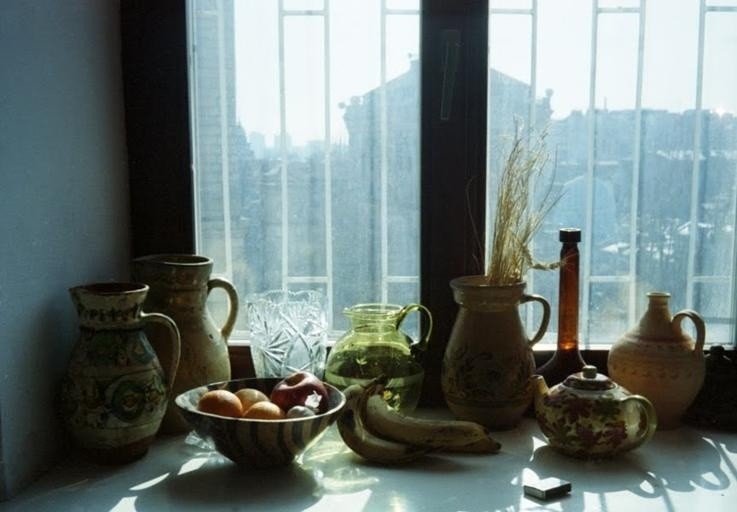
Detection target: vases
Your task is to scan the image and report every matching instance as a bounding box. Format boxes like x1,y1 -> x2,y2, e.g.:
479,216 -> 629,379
132,252 -> 239,432
607,291 -> 707,431
440,274 -> 550,432
58,282 -> 182,464
247,288 -> 332,382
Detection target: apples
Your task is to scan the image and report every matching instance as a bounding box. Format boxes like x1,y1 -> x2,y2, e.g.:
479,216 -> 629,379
243,400 -> 286,419
197,390 -> 243,418
269,371 -> 329,413
236,387 -> 271,412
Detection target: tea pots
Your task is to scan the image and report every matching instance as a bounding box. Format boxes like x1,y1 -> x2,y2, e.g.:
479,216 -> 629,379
528,366 -> 658,460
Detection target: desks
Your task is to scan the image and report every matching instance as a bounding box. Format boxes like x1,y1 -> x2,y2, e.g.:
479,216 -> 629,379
1,408 -> 736,511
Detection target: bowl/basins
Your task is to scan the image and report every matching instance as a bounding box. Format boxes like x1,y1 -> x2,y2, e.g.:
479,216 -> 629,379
174,377 -> 347,468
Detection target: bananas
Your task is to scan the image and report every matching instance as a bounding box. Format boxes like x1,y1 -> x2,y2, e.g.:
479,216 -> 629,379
337,373 -> 502,467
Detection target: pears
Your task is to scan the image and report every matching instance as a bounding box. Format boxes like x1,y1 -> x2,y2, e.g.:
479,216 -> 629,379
288,405 -> 316,418
